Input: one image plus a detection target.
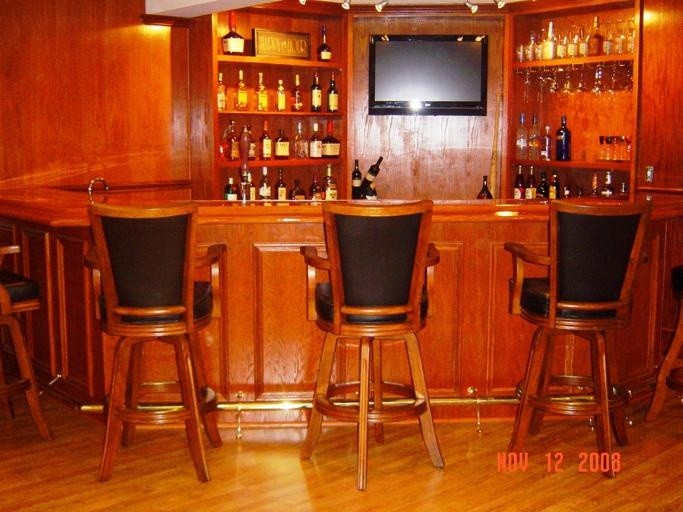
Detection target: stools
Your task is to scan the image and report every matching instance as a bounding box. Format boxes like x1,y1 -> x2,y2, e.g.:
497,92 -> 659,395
641,264 -> 683,425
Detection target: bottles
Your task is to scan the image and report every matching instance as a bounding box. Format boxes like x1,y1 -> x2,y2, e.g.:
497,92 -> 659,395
326,71 -> 339,113
216,71 -> 226,112
234,69 -> 247,111
274,168 -> 286,204
527,113 -> 540,160
511,164 -> 524,199
356,156 -> 383,192
359,182 -> 377,201
513,113 -> 527,160
253,70 -> 268,113
514,15 -> 635,64
238,160 -> 250,207
561,184 -> 571,199
309,71 -> 322,112
600,170 -> 613,197
273,129 -> 290,160
289,72 -> 303,113
224,120 -> 238,160
320,115 -> 340,158
589,171 -> 599,197
238,123 -> 248,161
547,171 -> 559,200
222,176 -> 238,200
619,182 -> 626,195
308,174 -> 323,205
245,123 -> 256,160
315,23 -> 332,61
221,9 -> 244,54
534,172 -> 547,199
292,121 -> 308,159
322,163 -> 337,201
309,121 -> 320,158
257,165 -> 271,206
475,175 -> 492,199
554,116 -> 570,160
275,79 -> 285,112
538,125 -> 552,161
246,172 -> 255,204
350,159 -> 361,200
288,179 -> 305,205
573,184 -> 582,197
523,164 -> 537,200
258,120 -> 271,161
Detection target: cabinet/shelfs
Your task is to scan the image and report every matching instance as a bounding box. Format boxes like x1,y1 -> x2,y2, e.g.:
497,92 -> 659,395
502,0 -> 682,198
170,2 -> 347,200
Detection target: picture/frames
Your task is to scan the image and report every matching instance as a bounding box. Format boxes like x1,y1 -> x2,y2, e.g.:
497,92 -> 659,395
252,26 -> 310,61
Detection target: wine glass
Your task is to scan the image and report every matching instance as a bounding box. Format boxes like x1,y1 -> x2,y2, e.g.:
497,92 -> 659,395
596,134 -> 631,162
521,59 -> 634,102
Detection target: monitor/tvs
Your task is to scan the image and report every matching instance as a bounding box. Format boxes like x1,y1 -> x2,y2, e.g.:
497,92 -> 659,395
368,33 -> 488,116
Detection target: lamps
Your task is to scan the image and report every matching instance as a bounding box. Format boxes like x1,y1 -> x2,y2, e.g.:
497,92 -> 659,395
300,1 -> 507,16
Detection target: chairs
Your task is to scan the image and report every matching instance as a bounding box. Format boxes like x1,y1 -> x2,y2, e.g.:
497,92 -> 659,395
0,244 -> 51,441
299,200 -> 446,491
502,201 -> 651,478
82,203 -> 227,484
